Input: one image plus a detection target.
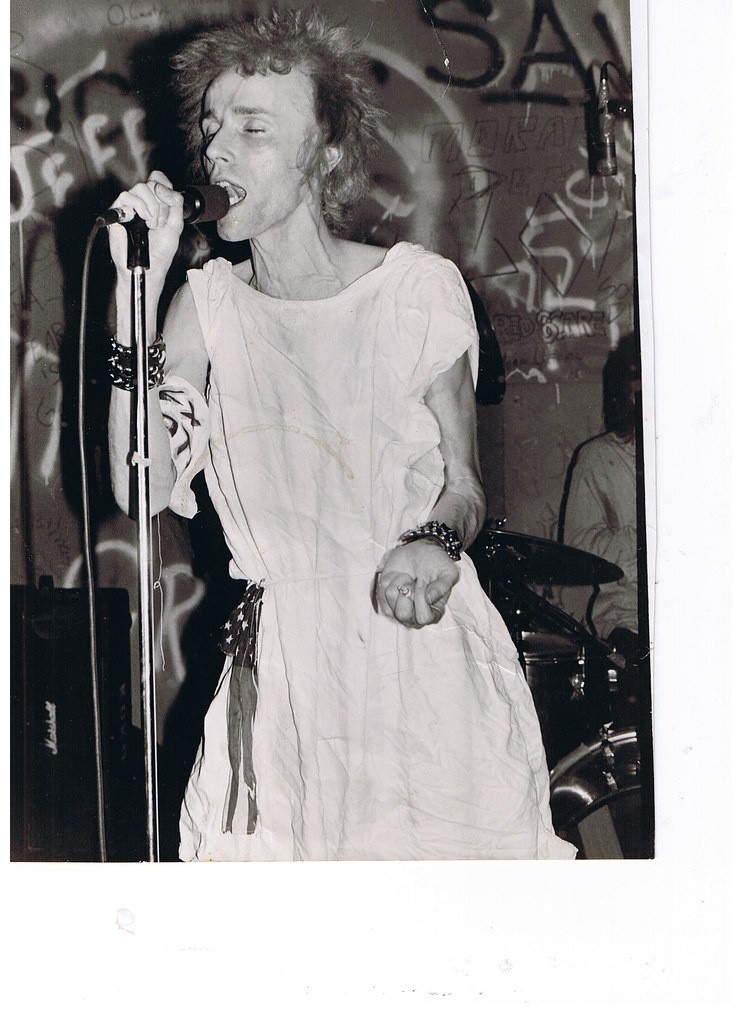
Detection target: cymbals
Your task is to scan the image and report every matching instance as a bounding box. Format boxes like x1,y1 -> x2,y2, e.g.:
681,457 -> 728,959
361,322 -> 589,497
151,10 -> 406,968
464,525 -> 626,588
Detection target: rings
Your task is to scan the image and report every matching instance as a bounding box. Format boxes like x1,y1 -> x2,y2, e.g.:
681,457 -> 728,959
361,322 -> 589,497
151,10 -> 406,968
430,605 -> 443,616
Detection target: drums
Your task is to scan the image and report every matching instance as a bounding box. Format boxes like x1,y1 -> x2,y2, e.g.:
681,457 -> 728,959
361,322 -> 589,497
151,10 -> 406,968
548,712 -> 652,838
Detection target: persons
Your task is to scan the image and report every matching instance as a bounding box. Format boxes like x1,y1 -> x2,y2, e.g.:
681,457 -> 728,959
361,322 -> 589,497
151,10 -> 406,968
553,331 -> 654,860
105,0 -> 580,860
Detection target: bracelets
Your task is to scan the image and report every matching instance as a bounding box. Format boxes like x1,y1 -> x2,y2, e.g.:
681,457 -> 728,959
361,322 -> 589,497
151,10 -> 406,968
106,331 -> 166,393
399,519 -> 463,562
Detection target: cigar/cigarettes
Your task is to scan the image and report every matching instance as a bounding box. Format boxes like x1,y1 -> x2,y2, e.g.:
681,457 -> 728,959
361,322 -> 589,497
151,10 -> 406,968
397,585 -> 414,601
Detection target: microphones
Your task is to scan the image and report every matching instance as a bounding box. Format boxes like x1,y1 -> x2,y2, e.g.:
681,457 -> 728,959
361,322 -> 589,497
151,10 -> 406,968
97,185 -> 230,228
597,66 -> 617,176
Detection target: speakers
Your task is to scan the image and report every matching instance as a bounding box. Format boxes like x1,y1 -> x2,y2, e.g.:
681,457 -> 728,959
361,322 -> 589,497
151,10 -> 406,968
10,582 -> 134,862
